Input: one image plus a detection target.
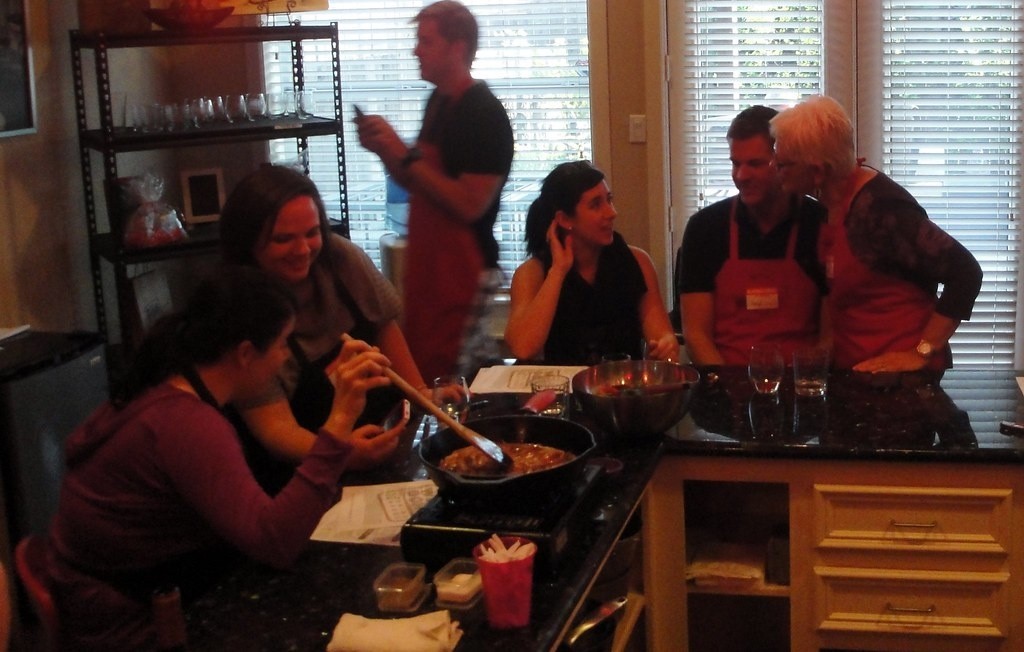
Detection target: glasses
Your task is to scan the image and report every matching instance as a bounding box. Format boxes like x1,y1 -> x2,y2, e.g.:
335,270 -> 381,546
772,148 -> 804,168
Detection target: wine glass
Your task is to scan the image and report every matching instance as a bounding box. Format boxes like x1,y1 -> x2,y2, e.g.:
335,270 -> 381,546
432,375 -> 470,424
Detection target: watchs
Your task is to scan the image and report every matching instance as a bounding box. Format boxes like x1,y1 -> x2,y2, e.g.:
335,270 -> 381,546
916,340 -> 940,360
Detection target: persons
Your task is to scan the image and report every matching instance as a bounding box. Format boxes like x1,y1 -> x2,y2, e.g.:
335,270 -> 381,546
355,1 -> 515,389
505,161 -> 681,364
769,97 -> 984,381
674,106 -> 828,366
53,163 -> 472,652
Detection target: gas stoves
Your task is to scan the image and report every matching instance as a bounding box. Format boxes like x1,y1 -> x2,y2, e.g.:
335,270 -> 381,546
397,462 -> 602,575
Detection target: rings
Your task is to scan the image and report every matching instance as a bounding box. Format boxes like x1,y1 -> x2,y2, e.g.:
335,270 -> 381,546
546,238 -> 550,243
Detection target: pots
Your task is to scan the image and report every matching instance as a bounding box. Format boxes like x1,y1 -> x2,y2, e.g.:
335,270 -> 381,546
419,414 -> 596,504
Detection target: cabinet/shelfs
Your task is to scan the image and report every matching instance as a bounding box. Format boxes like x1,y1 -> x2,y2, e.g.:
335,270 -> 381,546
560,451 -> 1024,652
70,18 -> 352,352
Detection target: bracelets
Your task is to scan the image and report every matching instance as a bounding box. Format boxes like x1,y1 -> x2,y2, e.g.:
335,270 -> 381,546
399,147 -> 424,168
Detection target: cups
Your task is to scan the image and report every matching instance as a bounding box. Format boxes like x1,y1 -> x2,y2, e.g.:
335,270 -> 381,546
601,352 -> 631,363
473,537 -> 537,628
529,374 -> 569,417
748,345 -> 786,394
130,89 -> 315,132
793,347 -> 830,397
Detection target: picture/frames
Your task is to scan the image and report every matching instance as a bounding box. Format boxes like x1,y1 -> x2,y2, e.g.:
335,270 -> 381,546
0,0 -> 39,138
179,167 -> 226,223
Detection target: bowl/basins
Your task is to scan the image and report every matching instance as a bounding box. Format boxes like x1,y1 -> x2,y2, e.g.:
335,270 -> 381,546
146,6 -> 234,31
572,359 -> 701,438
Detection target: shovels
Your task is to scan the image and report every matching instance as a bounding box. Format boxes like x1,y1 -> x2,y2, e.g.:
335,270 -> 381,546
341,334 -> 514,471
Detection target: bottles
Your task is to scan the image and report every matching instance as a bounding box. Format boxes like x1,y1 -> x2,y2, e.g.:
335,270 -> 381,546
138,562 -> 187,646
384,163 -> 408,237
